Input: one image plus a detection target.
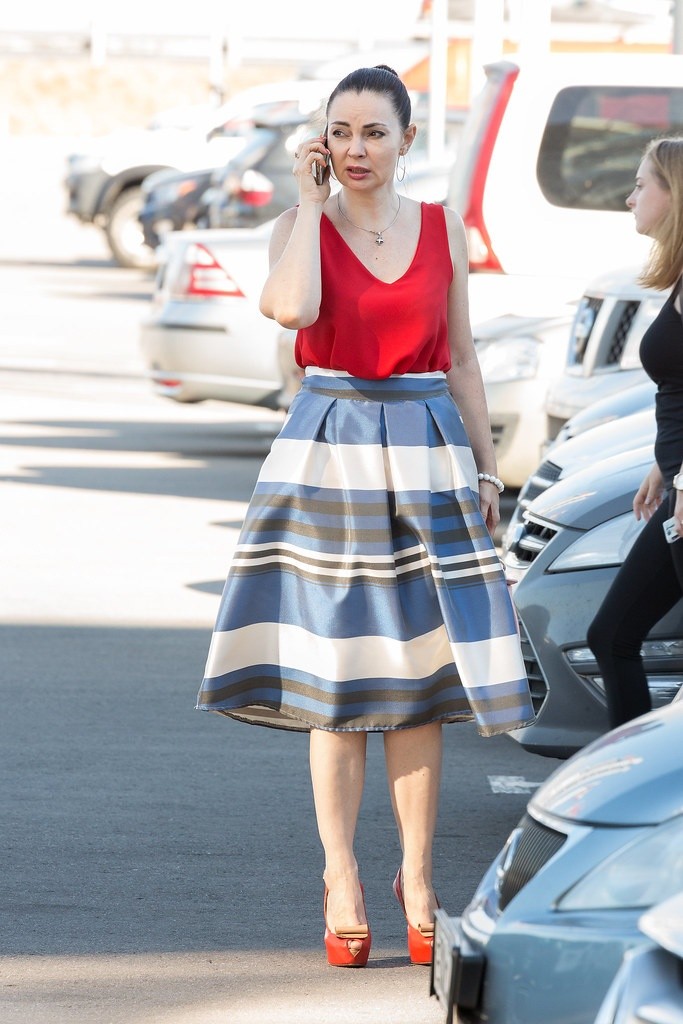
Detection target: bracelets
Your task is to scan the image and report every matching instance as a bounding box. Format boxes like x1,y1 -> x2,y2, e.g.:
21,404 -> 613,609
478,473 -> 504,494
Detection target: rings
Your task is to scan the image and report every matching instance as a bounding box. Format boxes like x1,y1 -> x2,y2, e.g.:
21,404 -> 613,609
295,153 -> 299,158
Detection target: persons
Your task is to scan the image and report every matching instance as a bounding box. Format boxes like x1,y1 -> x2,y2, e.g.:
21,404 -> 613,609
193,64 -> 538,968
585,137 -> 683,729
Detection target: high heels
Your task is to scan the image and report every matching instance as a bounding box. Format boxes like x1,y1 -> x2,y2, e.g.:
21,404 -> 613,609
324,880 -> 371,966
393,868 -> 443,964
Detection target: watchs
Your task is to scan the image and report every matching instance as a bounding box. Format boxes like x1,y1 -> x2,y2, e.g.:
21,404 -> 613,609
673,472 -> 683,490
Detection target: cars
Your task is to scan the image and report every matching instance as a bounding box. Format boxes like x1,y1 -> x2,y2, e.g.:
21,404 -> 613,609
427,266 -> 683,1024
147,162 -> 454,416
59,56 -> 434,273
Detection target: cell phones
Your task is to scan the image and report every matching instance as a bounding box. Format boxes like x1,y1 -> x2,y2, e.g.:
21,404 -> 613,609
663,516 -> 681,544
315,127 -> 329,185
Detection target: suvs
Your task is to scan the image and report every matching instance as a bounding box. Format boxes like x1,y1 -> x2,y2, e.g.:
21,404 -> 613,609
451,54 -> 683,488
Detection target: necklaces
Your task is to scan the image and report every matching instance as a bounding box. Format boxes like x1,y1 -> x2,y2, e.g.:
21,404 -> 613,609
337,192 -> 401,245
